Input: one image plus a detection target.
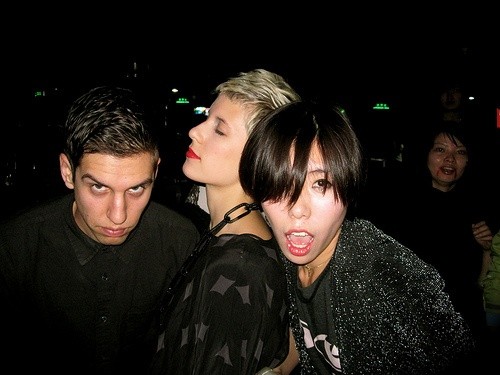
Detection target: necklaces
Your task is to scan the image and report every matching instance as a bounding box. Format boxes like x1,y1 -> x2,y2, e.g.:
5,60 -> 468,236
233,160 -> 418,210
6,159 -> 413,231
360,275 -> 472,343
304,258 -> 330,277
155,202 -> 260,336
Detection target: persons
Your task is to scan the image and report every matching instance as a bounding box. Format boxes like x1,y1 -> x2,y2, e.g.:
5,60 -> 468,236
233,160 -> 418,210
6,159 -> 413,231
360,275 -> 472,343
483,229 -> 500,345
137,68 -> 301,375
431,72 -> 482,134
0,86 -> 203,375
387,116 -> 492,341
238,103 -> 476,375
